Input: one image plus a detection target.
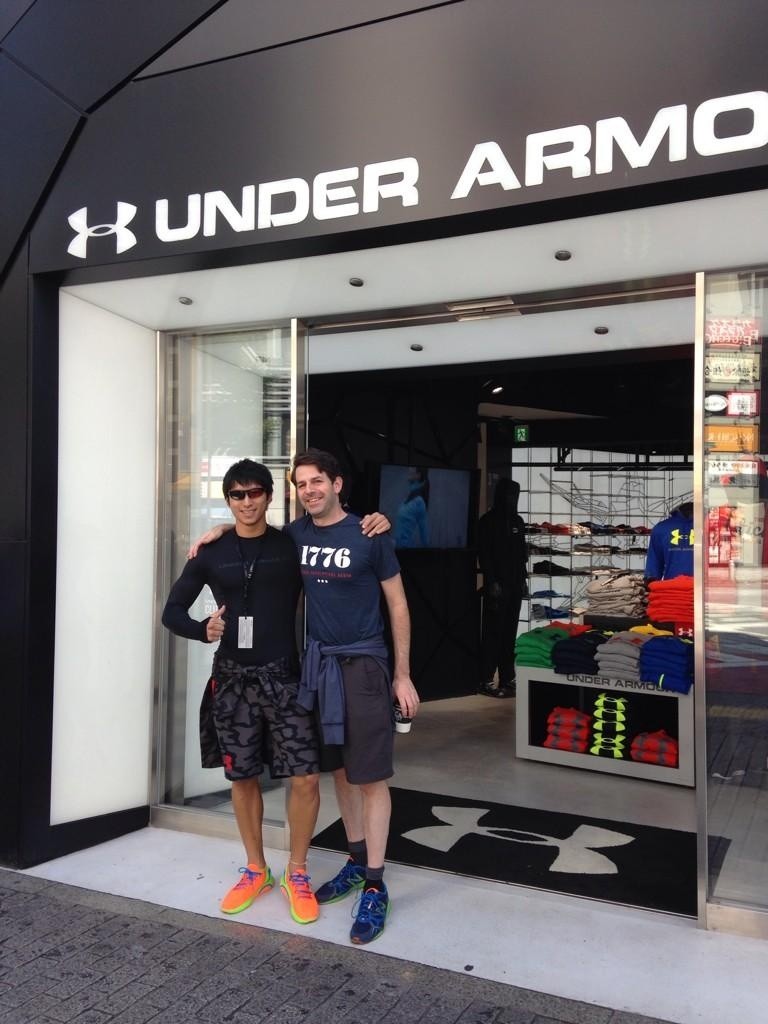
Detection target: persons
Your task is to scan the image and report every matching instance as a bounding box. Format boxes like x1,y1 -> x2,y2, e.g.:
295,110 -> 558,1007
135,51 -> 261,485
185,448 -> 420,946
161,459 -> 391,924
394,466 -> 430,549
469,479 -> 529,698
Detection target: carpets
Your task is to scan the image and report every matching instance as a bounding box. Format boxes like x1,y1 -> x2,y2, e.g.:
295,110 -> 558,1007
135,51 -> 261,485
309,786 -> 731,920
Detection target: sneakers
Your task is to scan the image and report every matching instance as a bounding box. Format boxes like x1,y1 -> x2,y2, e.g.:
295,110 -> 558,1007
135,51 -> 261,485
314,857 -> 368,905
219,863 -> 275,914
349,880 -> 391,946
279,865 -> 320,924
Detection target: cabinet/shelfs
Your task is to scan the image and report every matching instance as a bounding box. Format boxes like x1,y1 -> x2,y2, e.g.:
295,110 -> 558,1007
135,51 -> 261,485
519,533 -> 651,633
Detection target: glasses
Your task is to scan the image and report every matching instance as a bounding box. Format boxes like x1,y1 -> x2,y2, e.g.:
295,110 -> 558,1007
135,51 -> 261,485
229,488 -> 267,500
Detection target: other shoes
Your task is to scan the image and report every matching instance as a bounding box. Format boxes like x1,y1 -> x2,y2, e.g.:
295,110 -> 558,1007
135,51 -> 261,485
480,680 -> 504,697
499,677 -> 516,693
